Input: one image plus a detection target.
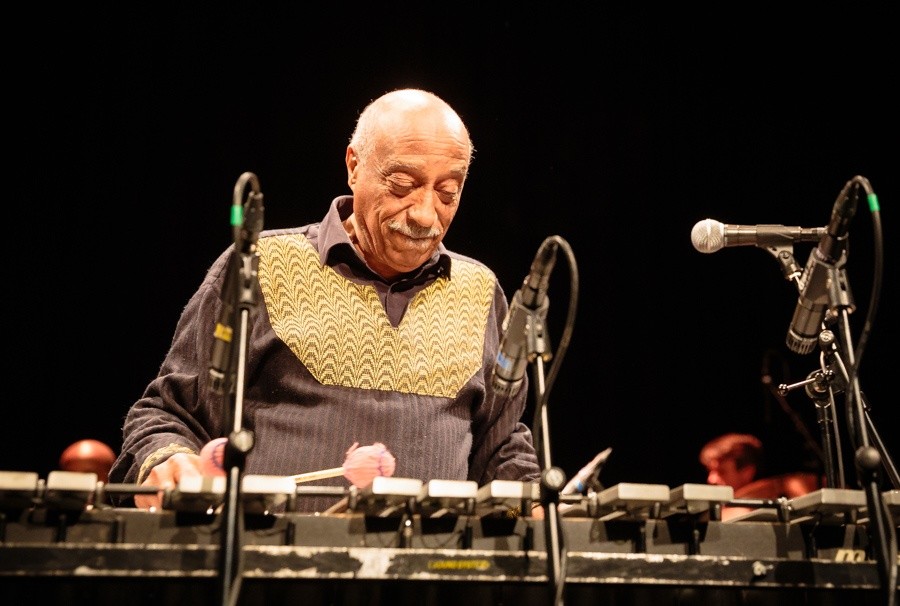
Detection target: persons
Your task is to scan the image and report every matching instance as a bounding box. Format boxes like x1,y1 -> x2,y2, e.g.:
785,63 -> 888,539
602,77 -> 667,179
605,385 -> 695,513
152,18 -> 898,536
703,435 -> 794,524
103,89 -> 548,520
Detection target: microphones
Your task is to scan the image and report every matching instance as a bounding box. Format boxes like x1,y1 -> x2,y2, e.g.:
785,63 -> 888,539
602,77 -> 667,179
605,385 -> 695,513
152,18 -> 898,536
785,182 -> 863,355
207,193 -> 263,395
692,218 -> 825,253
491,240 -> 559,396
